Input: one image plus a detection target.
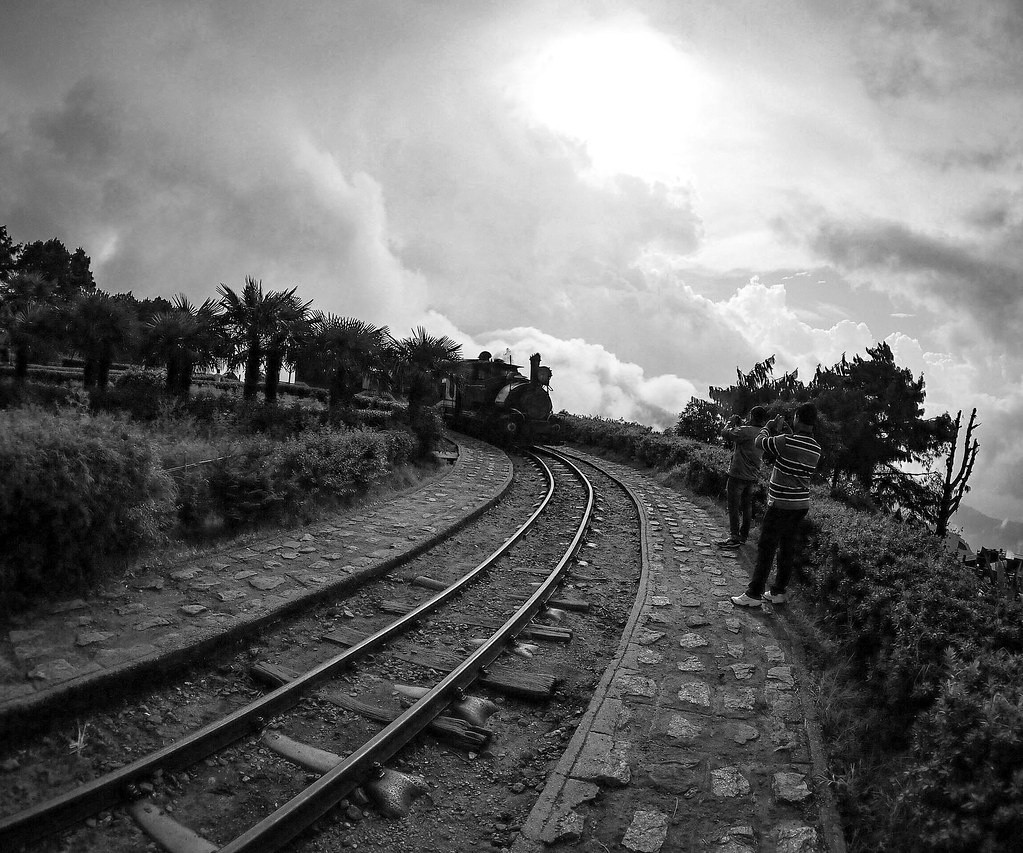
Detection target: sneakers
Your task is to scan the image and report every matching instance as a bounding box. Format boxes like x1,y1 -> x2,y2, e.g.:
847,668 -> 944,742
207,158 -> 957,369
763,591 -> 784,604
718,539 -> 740,548
731,592 -> 762,607
738,535 -> 746,544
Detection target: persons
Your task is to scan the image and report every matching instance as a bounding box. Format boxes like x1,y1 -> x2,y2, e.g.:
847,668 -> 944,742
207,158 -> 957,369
729,403 -> 822,607
717,406 -> 767,549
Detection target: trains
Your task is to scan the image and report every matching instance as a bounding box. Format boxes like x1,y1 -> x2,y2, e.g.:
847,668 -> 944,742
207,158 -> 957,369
433,351 -> 561,449
